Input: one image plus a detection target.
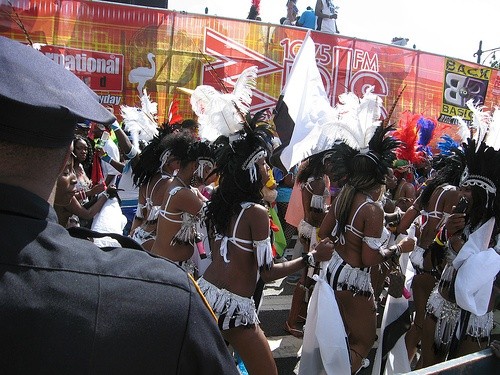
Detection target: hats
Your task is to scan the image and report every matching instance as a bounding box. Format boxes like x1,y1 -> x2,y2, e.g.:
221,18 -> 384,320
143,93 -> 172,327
0,36 -> 116,148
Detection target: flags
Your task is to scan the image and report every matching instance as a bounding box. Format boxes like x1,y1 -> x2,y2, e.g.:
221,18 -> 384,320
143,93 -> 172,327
269,33 -> 337,177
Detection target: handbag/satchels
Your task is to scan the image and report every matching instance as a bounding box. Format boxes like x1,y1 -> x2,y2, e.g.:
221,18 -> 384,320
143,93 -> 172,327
387,273 -> 405,298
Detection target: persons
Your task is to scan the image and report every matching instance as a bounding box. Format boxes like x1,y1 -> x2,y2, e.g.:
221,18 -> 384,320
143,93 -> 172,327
246,0 -> 340,33
52,107 -> 500,375
1,36 -> 240,375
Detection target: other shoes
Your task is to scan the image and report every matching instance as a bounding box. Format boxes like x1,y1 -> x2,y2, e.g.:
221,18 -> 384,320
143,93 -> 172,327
281,320 -> 304,338
296,314 -> 306,322
